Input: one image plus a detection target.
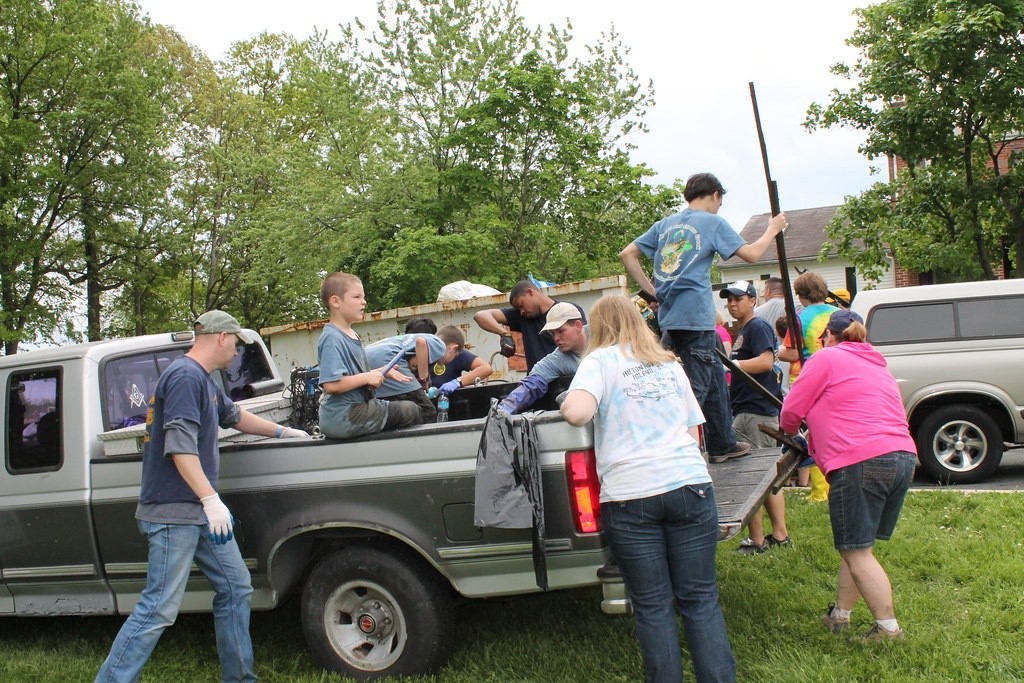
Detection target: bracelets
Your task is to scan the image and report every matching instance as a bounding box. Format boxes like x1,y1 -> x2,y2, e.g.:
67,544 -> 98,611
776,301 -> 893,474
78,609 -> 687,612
418,375 -> 429,383
276,424 -> 282,437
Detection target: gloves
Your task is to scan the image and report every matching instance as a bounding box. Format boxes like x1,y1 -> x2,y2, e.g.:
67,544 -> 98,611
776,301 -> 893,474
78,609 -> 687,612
500,333 -> 516,357
438,379 -> 461,395
200,493 -> 235,544
280,426 -> 310,438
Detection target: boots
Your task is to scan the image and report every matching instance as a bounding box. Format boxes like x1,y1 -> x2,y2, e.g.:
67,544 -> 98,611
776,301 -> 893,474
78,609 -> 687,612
802,466 -> 830,501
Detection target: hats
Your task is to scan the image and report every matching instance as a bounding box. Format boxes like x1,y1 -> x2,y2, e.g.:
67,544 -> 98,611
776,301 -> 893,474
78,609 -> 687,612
539,301 -> 582,335
825,289 -> 851,303
720,280 -> 757,298
818,310 -> 863,340
194,309 -> 254,344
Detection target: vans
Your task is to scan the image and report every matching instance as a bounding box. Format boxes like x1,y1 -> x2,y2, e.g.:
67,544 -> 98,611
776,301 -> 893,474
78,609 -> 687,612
850,278 -> 1024,485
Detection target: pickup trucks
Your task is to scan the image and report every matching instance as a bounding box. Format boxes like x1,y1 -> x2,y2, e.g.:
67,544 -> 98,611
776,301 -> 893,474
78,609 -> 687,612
0,328 -> 809,683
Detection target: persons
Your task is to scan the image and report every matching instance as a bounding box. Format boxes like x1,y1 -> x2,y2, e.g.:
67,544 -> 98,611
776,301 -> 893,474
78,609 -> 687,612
637,271 -> 852,555
402,317 -> 494,396
364,323 -> 466,423
316,274 -> 422,438
471,282 -> 587,377
94,310 -> 309,682
497,302 -> 595,415
553,292 -> 740,683
781,308 -> 918,642
619,171 -> 788,465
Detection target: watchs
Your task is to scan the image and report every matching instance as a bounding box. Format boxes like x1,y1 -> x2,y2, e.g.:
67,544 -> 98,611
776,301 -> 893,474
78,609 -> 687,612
456,378 -> 462,388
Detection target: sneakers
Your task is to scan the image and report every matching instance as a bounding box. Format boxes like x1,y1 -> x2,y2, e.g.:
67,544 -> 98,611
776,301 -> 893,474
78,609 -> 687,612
731,537 -> 769,556
709,441 -> 752,463
848,621 -> 904,645
764,534 -> 791,546
822,602 -> 850,633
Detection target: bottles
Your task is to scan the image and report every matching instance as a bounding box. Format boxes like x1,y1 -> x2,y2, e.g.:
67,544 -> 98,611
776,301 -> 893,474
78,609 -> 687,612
424,387 -> 440,399
437,391 -> 449,423
